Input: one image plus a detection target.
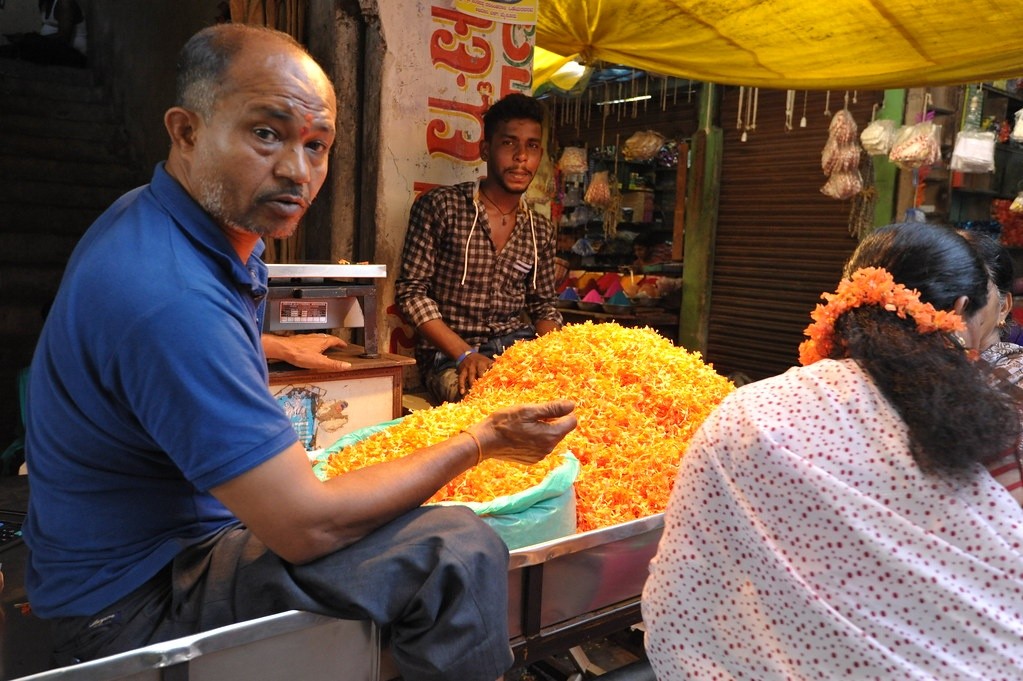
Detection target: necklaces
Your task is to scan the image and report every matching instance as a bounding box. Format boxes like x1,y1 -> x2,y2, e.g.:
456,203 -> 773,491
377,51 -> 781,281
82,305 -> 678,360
480,189 -> 519,226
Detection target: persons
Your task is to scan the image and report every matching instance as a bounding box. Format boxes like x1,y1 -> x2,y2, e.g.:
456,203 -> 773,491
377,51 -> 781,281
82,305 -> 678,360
395,92 -> 564,406
642,221 -> 1023,681
21,24 -> 578,681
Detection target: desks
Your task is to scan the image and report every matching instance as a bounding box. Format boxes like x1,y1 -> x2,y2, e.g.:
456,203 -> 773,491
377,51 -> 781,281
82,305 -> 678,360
257,343 -> 416,461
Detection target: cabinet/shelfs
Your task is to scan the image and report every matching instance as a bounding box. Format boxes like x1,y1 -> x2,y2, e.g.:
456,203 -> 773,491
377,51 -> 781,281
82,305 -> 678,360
557,151 -> 689,345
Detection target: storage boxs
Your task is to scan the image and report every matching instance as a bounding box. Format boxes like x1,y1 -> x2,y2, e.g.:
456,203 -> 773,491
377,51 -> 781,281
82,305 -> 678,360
920,84 -> 1023,302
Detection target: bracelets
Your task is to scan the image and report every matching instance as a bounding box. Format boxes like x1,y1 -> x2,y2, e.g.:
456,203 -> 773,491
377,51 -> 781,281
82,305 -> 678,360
460,430 -> 483,466
456,349 -> 477,368
